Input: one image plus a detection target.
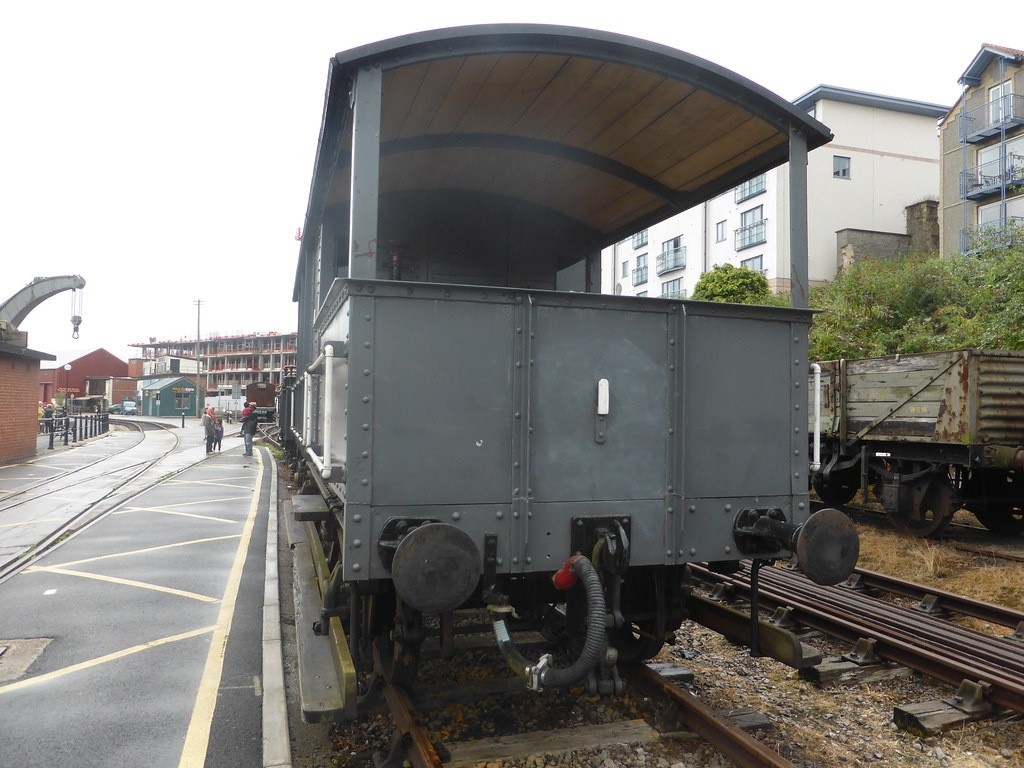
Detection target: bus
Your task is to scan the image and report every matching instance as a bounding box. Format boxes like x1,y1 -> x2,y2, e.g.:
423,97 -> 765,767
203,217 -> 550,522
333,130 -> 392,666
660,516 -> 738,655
67,398 -> 100,413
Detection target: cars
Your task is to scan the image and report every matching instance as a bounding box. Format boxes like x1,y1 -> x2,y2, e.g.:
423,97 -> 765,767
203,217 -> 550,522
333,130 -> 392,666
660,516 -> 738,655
119,400 -> 138,414
108,404 -> 122,414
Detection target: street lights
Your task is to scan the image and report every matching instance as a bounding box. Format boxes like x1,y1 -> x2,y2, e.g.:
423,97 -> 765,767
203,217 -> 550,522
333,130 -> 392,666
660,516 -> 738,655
63,363 -> 71,407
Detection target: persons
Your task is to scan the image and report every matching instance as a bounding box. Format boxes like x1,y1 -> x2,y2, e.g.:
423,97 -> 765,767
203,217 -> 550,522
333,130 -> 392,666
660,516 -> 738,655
37,400 -> 53,433
240,401 -> 257,456
201,407 -> 217,454
212,418 -> 223,451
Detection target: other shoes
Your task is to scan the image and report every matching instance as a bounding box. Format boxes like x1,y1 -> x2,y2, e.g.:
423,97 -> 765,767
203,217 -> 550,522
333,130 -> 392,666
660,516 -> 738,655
218,451 -> 221,454
206,450 -> 216,454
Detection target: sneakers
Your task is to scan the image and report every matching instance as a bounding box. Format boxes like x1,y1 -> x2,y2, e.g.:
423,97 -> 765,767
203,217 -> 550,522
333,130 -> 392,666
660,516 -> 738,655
239,430 -> 243,436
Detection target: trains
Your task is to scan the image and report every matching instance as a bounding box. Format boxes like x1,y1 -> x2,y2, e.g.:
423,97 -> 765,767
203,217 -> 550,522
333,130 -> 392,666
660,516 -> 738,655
271,18 -> 839,706
245,381 -> 276,420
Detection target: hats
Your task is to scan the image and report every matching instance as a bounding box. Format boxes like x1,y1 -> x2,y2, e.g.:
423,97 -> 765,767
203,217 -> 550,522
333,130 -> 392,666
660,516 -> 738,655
249,402 -> 256,406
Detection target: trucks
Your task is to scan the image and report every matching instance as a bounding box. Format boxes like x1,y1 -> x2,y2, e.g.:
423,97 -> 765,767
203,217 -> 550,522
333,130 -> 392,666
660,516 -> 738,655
806,342 -> 1024,543
